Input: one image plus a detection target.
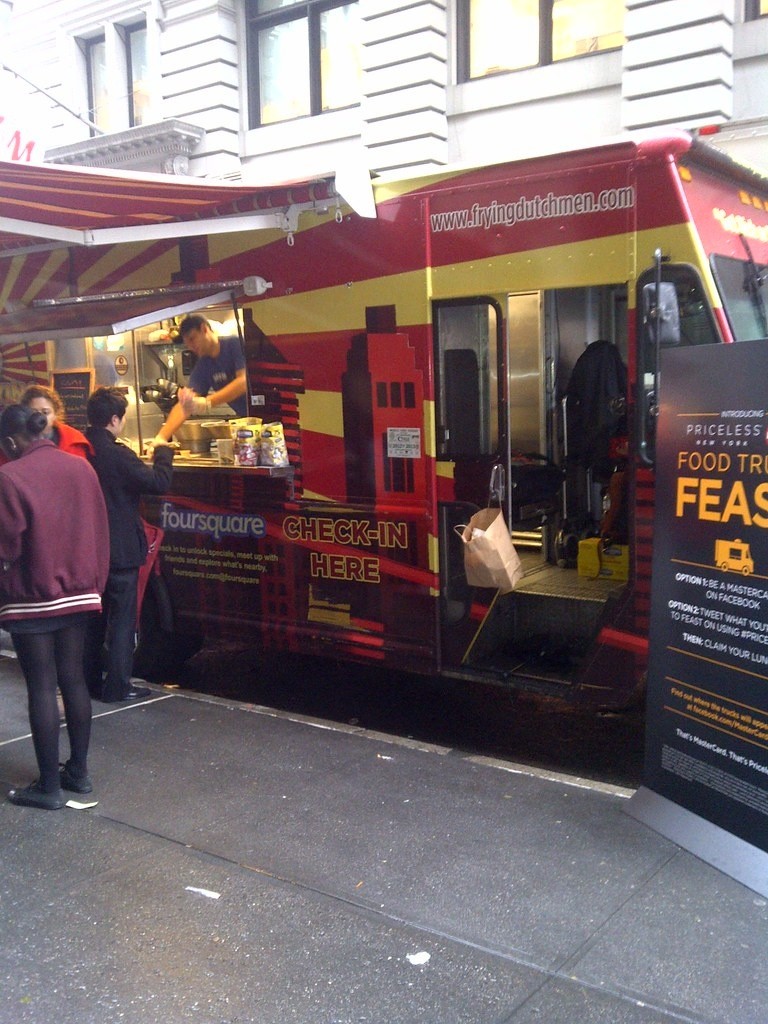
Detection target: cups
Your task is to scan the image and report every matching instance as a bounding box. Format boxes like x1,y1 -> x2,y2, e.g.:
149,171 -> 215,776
216,438 -> 235,465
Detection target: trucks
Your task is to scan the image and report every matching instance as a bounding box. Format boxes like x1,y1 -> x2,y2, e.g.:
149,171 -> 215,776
0,130 -> 768,739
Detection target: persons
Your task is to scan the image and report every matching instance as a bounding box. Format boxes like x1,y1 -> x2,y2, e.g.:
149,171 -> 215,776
146,314 -> 248,460
0,383 -> 96,721
1,402 -> 110,811
83,383 -> 176,704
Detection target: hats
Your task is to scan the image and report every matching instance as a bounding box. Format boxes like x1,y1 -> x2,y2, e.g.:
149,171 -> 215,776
172,315 -> 205,344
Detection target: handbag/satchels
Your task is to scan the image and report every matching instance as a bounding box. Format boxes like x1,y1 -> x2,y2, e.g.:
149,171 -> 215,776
453,463 -> 525,589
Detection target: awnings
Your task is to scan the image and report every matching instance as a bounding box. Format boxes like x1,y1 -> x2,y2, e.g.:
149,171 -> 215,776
0,154 -> 375,258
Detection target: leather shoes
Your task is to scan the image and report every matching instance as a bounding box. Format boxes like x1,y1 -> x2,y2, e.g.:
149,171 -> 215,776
6,770 -> 99,810
102,686 -> 151,703
